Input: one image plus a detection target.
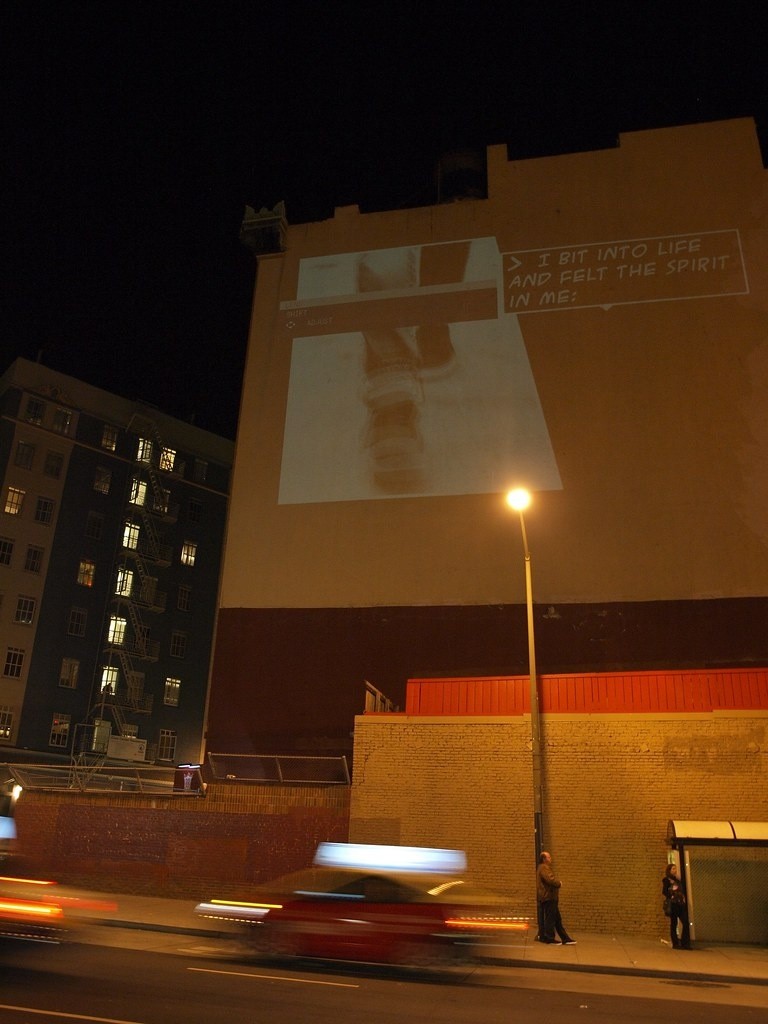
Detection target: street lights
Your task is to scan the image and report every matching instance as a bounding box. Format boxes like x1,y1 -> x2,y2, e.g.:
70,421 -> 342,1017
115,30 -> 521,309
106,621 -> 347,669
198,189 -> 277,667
504,485 -> 546,945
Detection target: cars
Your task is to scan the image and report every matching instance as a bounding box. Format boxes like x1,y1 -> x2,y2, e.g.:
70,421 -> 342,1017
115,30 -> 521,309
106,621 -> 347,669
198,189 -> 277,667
183,839 -> 529,984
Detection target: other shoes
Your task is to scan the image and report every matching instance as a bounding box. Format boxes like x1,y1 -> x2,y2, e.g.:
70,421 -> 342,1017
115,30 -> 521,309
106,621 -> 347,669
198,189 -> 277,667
549,939 -> 562,945
563,938 -> 577,944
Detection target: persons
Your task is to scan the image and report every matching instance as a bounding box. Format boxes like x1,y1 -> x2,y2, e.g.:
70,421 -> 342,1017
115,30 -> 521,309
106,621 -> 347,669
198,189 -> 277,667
661,863 -> 692,950
535,851 -> 578,945
103,683 -> 113,694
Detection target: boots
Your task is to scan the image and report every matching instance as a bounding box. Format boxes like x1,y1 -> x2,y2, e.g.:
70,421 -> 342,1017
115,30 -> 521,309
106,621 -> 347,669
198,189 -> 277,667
680,940 -> 692,950
672,940 -> 684,949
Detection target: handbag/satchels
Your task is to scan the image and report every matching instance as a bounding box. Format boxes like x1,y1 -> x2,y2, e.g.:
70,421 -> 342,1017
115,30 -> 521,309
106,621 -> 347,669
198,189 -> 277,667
662,899 -> 672,917
